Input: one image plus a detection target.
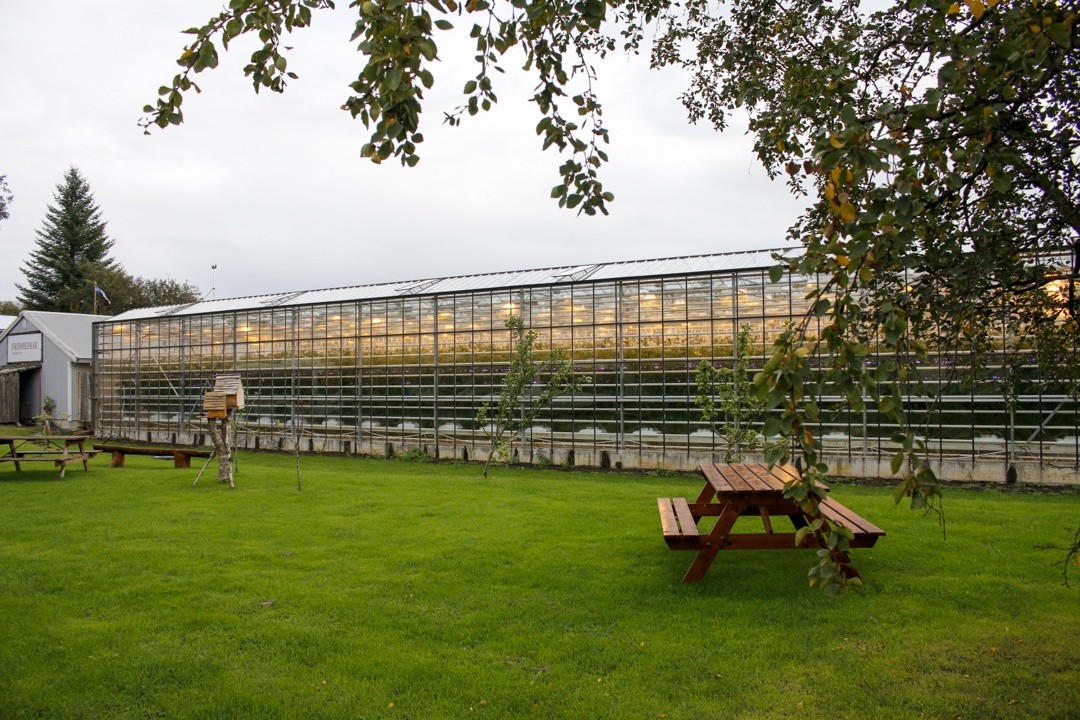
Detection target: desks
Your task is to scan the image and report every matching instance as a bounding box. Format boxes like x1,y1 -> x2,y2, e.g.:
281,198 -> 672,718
680,464 -> 861,584
0,436 -> 90,478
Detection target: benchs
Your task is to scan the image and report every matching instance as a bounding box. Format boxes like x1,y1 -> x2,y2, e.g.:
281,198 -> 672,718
655,496 -> 711,552
92,443 -> 213,470
7,451 -> 103,459
818,495 -> 888,548
0,458 -> 68,468
